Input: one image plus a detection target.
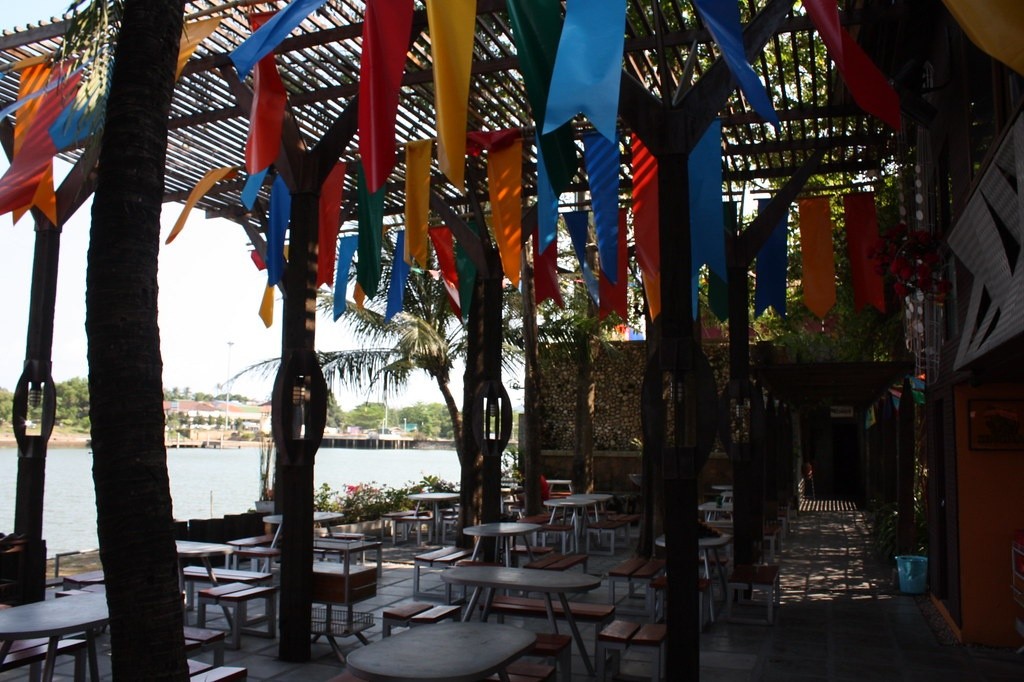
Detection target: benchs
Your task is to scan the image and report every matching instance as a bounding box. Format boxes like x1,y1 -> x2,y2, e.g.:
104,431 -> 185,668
1,490 -> 794,682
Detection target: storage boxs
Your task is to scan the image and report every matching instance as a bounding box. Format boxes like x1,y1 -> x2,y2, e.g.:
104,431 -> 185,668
312,562 -> 378,605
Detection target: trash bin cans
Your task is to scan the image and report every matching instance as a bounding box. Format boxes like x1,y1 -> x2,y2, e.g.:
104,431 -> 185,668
894,555 -> 929,595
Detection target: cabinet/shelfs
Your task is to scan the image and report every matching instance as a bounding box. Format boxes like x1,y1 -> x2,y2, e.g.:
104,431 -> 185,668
311,533 -> 382,662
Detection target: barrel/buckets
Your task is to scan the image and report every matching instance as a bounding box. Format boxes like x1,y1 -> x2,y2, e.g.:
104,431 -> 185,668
895,555 -> 927,593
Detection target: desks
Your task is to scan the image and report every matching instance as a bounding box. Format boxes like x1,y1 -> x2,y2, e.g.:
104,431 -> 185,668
0,477 -> 795,682
